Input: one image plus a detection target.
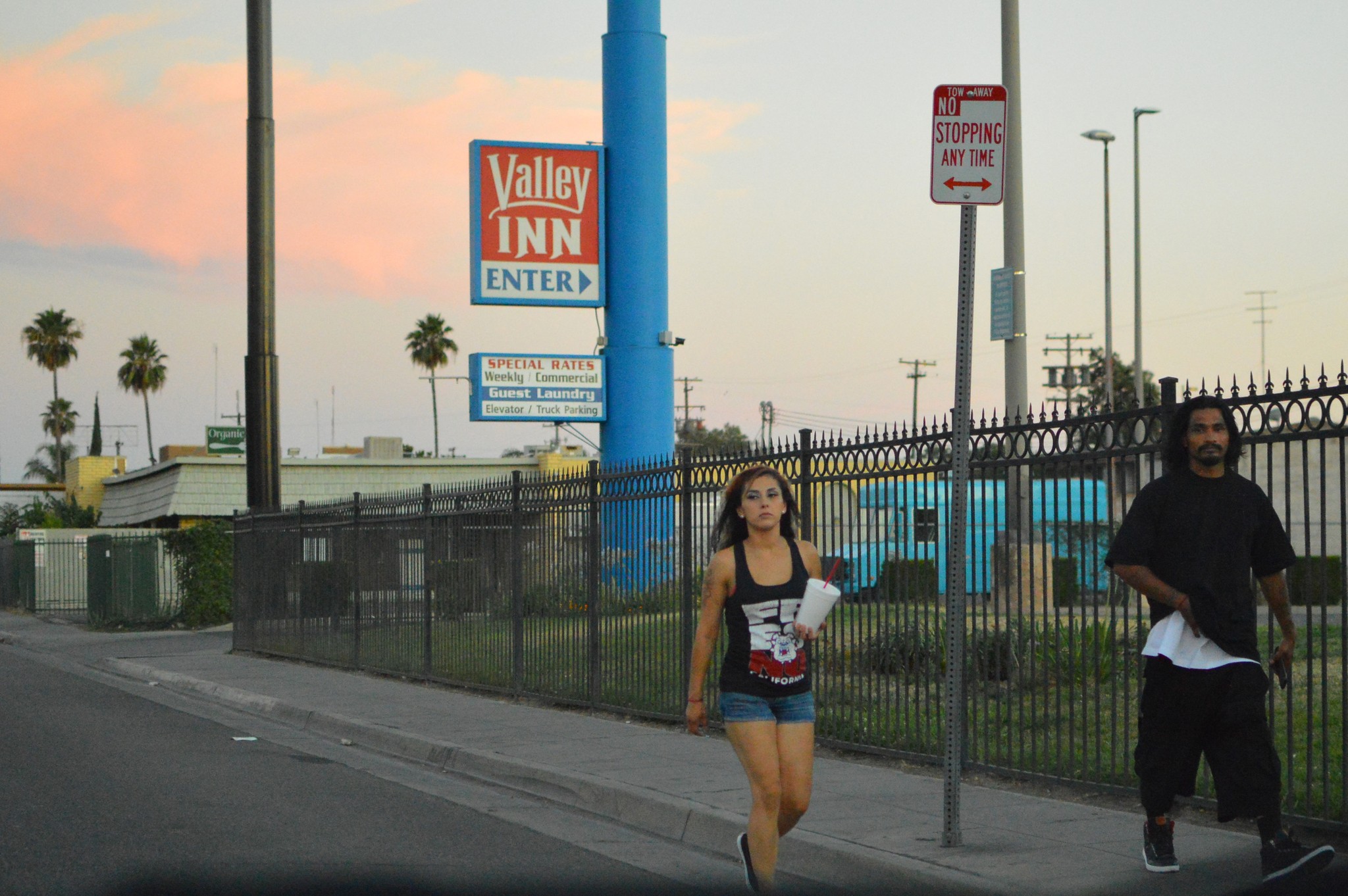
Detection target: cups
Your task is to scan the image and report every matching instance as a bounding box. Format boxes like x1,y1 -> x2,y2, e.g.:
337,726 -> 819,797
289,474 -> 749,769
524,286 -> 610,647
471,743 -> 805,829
795,578 -> 841,636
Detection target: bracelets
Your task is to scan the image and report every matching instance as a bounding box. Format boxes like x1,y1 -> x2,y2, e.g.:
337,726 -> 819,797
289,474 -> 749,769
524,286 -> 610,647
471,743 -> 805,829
687,698 -> 704,704
1177,596 -> 1188,610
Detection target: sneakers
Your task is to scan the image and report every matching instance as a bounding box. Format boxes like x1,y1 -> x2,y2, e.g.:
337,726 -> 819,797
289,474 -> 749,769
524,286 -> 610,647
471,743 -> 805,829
736,832 -> 760,895
1260,824 -> 1337,889
1141,814 -> 1181,873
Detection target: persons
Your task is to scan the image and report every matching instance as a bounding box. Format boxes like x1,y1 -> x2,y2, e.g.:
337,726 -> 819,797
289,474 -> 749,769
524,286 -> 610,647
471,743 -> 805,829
682,465 -> 824,896
1103,393 -> 1338,893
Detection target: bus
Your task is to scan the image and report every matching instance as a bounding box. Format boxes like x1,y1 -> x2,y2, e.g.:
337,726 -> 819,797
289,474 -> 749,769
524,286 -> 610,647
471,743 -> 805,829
822,477 -> 1114,603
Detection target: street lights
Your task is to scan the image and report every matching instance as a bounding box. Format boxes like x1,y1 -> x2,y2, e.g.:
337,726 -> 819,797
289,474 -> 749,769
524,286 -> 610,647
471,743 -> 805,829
1079,104 -> 1159,610
